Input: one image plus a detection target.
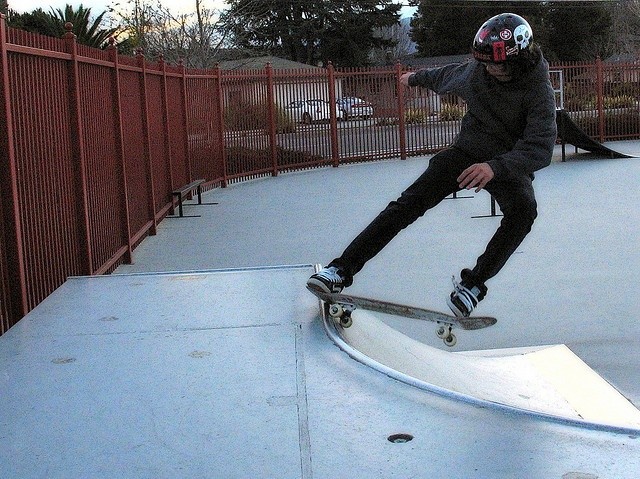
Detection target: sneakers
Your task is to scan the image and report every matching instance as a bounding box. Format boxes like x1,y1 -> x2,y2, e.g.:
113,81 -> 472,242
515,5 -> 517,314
306,266 -> 347,295
446,275 -> 487,318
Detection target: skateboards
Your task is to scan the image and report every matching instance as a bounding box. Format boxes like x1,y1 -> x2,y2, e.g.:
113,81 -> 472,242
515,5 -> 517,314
305,286 -> 497,347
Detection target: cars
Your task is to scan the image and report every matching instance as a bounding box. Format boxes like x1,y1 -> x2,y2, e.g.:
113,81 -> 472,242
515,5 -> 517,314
280,98 -> 344,124
327,97 -> 376,120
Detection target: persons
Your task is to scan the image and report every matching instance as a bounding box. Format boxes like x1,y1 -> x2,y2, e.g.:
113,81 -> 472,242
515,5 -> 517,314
305,11 -> 557,320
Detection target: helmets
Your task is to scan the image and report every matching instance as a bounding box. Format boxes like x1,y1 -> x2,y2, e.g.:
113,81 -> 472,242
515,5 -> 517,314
470,12 -> 534,64
